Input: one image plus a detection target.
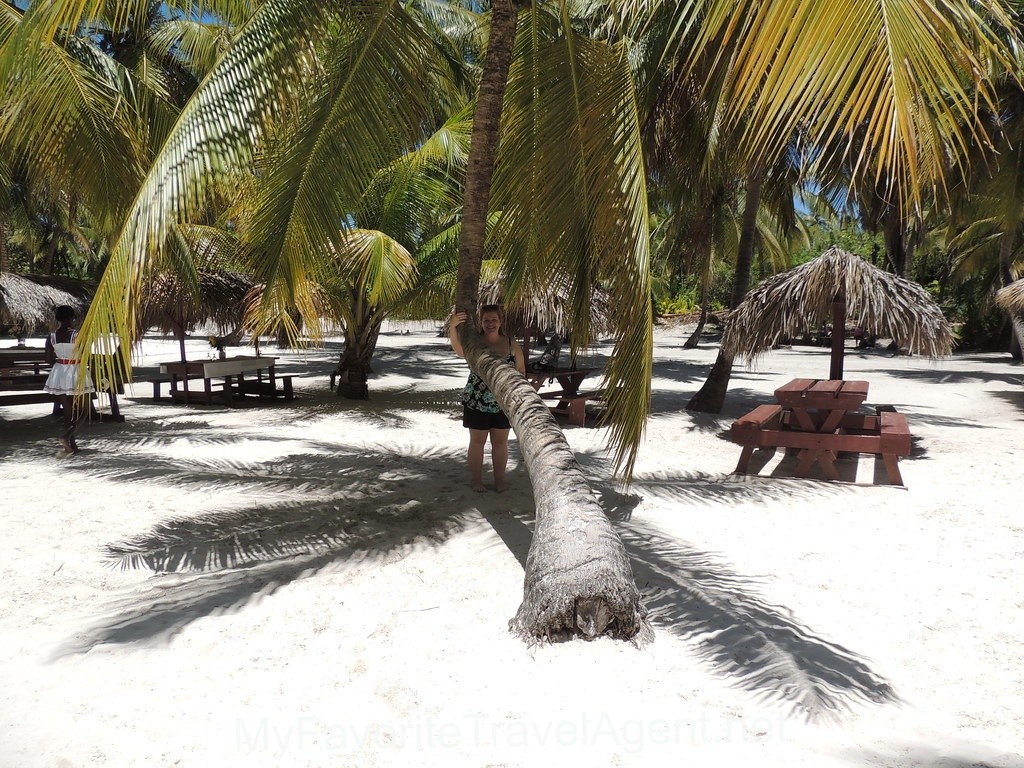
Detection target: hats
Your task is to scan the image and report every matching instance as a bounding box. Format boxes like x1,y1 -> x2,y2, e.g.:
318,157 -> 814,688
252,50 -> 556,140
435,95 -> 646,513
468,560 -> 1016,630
55,306 -> 77,320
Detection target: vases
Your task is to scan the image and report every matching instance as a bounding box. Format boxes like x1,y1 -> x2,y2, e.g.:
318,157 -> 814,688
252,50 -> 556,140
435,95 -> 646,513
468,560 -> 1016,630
219,349 -> 226,359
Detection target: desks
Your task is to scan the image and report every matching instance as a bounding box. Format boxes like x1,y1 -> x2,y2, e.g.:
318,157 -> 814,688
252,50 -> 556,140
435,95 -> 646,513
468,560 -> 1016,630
759,378 -> 880,480
527,367 -> 606,415
0,352 -> 57,361
160,357 -> 280,405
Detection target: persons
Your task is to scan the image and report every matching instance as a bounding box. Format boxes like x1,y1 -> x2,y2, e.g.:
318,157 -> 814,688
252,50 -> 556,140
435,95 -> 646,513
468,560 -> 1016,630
449,304 -> 525,493
44,305 -> 95,453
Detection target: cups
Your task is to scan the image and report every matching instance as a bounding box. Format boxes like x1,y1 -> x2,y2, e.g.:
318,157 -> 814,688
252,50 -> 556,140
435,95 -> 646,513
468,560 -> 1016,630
160,366 -> 167,373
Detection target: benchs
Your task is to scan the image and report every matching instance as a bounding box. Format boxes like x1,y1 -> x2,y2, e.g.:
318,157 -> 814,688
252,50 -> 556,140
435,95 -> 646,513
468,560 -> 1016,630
731,405 -> 782,475
147,375 -> 204,402
539,391 -> 564,400
879,412 -> 912,486
0,365 -> 53,385
14,362 -> 47,375
558,387 -> 623,428
212,375 -> 299,408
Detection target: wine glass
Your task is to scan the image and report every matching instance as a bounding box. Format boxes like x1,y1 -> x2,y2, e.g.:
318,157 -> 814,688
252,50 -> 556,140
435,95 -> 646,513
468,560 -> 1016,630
206,352 -> 218,362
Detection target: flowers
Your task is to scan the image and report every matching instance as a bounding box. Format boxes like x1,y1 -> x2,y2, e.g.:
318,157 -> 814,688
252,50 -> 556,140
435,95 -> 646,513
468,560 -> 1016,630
208,336 -> 228,351
